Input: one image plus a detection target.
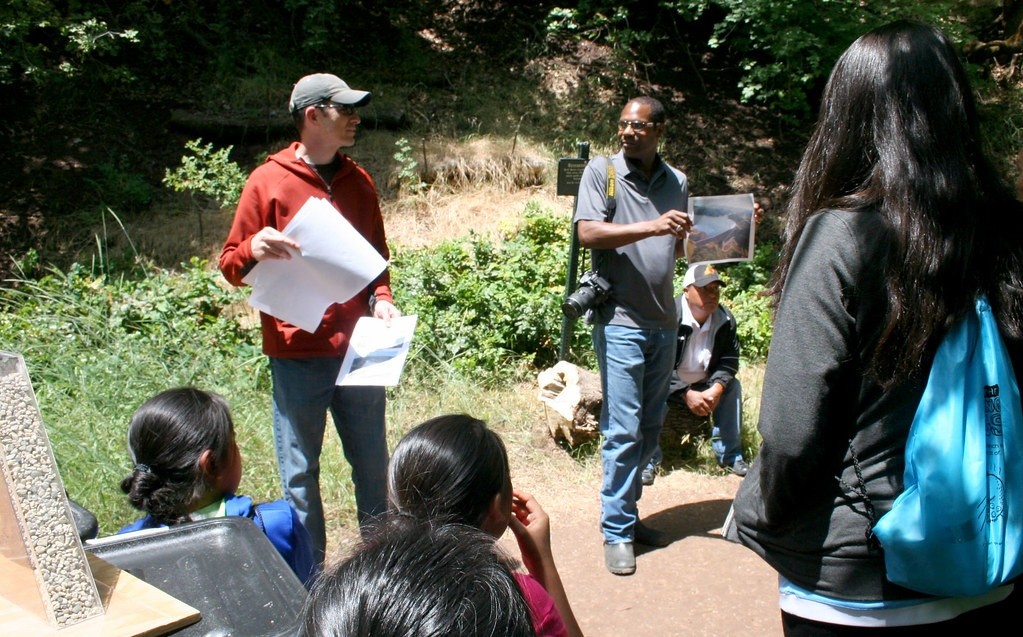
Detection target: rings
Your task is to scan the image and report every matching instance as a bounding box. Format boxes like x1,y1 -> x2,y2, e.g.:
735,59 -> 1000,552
676,225 -> 683,232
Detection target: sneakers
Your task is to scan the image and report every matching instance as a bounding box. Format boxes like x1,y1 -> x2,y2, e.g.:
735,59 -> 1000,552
634,520 -> 670,548
603,541 -> 636,575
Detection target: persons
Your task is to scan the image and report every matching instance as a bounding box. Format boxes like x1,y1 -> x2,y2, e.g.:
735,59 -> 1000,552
642,264 -> 750,484
721,19 -> 1023,637
219,72 -> 403,571
572,96 -> 766,576
118,387 -> 584,637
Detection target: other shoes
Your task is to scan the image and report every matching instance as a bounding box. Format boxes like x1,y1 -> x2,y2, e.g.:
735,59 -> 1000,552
721,460 -> 751,475
642,468 -> 655,484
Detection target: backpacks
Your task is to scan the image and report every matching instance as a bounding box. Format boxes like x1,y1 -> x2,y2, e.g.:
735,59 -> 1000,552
872,288 -> 1023,599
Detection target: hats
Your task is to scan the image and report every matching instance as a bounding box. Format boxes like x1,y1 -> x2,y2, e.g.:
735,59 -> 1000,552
288,73 -> 372,113
683,263 -> 727,288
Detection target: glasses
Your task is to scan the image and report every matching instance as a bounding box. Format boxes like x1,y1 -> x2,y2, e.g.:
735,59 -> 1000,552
617,120 -> 666,130
313,103 -> 356,115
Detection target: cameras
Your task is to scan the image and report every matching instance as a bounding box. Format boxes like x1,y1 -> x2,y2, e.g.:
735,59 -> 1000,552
559,267 -> 613,320
